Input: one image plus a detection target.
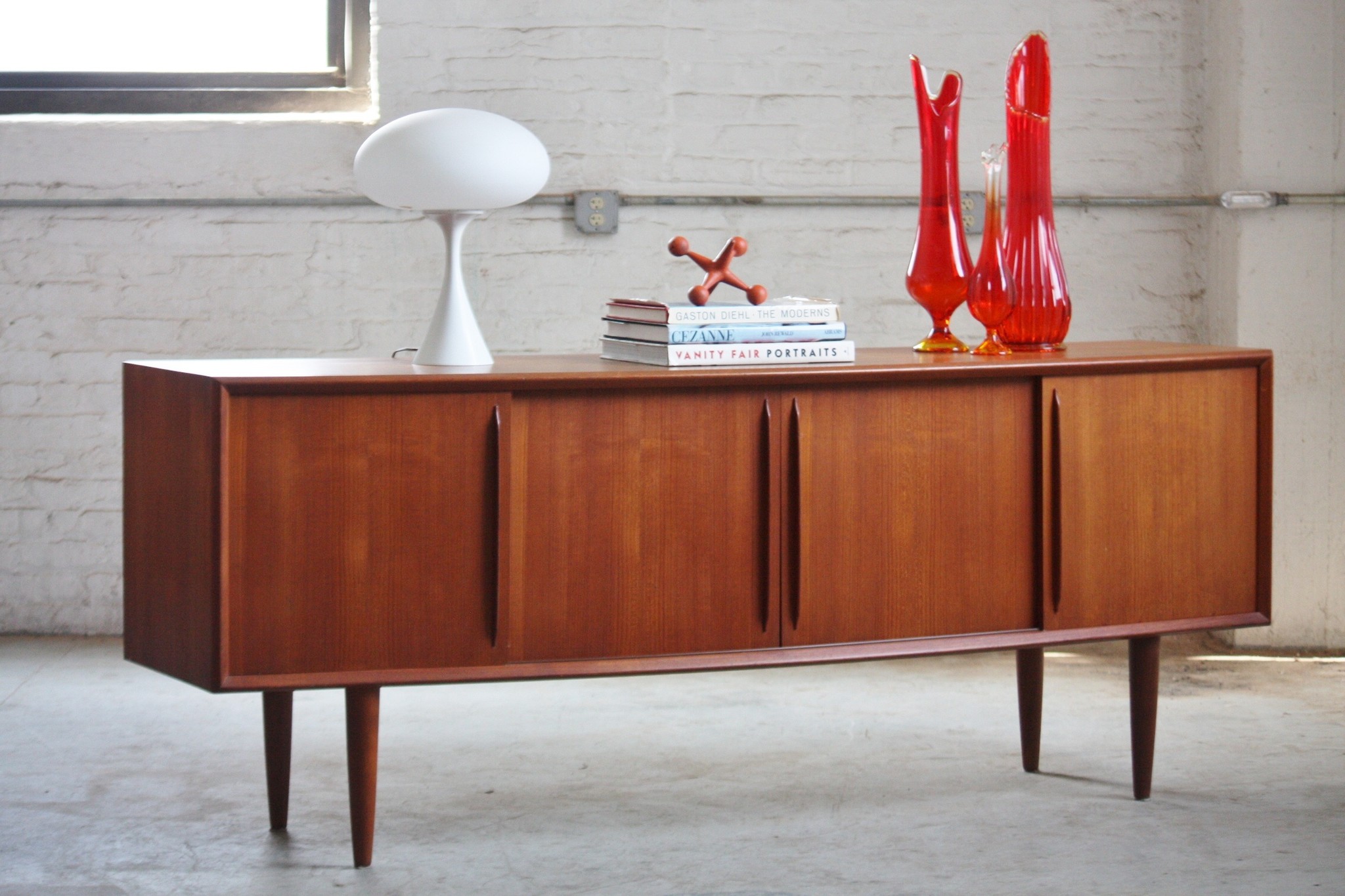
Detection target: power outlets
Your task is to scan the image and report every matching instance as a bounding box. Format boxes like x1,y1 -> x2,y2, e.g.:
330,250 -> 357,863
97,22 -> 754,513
573,189 -> 619,235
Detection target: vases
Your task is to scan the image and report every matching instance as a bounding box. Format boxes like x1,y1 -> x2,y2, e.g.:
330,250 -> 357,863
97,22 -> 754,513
908,30 -> 1071,357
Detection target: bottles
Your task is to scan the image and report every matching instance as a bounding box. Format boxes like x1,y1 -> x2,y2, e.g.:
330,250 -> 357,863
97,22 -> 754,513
909,28 -> 1072,355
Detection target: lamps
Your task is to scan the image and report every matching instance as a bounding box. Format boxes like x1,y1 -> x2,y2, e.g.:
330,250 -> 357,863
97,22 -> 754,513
352,106 -> 548,367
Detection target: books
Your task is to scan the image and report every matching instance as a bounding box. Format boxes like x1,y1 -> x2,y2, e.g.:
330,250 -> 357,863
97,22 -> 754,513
606,297 -> 839,324
602,317 -> 847,344
599,337 -> 855,367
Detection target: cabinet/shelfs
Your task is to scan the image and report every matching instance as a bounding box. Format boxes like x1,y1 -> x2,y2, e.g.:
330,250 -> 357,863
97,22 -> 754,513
119,339 -> 1275,869
